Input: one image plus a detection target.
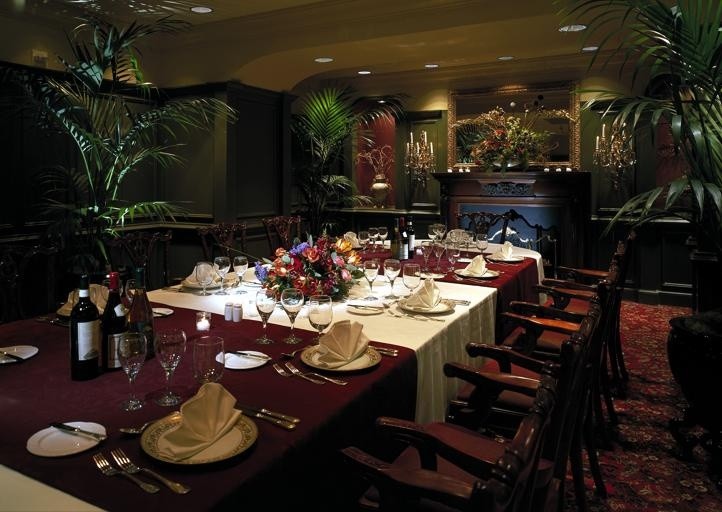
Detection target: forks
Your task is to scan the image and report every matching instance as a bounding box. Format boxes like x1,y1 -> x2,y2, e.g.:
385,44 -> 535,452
286,362 -> 326,386
271,363 -> 349,387
94,451 -> 160,496
110,447 -> 190,493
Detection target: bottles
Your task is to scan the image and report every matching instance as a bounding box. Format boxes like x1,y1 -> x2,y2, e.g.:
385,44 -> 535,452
71,273 -> 102,381
406,216 -> 415,259
131,267 -> 153,362
391,218 -> 402,261
400,217 -> 409,260
100,270 -> 129,373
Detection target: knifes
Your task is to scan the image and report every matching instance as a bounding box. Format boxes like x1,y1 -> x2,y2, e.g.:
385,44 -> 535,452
372,345 -> 401,355
239,411 -> 295,431
373,351 -> 398,359
45,420 -> 108,444
239,407 -> 300,425
223,347 -> 276,364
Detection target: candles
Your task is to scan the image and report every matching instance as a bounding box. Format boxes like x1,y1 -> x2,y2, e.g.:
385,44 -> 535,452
406,143 -> 410,157
595,137 -> 599,153
416,143 -> 420,157
610,136 -> 614,152
628,134 -> 633,153
429,141 -> 434,155
423,130 -> 428,148
602,123 -> 605,139
622,122 -> 625,140
410,132 -> 414,146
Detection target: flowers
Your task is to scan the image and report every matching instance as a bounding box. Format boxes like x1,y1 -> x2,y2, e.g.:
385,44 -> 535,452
449,96 -> 559,168
254,235 -> 367,304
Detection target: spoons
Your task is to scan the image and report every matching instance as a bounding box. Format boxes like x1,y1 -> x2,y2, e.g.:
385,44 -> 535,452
118,420 -> 156,435
280,347 -> 308,359
0,351 -> 27,364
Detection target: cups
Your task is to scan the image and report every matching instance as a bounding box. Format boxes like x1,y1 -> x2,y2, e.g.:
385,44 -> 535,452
281,288 -> 305,345
383,259 -> 401,299
449,231 -> 460,250
448,243 -> 463,280
378,227 -> 389,253
368,228 -> 378,254
233,255 -> 248,295
214,256 -> 230,295
194,336 -> 225,384
197,311 -> 211,333
363,260 -> 380,300
256,292 -> 277,345
402,264 -> 422,297
431,240 -> 447,272
428,223 -> 446,244
196,262 -> 213,297
118,333 -> 147,412
476,234 -> 488,257
462,230 -> 474,258
308,294 -> 333,342
153,329 -> 186,406
358,232 -> 369,254
421,241 -> 433,271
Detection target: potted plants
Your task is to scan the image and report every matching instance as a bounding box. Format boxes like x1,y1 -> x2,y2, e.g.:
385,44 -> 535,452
556,1 -> 722,418
354,144 -> 395,208
2,12 -> 239,286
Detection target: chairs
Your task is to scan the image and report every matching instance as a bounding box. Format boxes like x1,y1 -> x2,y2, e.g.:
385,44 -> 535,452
262,214 -> 303,258
466,279 -> 626,503
328,363 -> 566,507
197,222 -> 249,268
538,232 -> 636,395
373,305 -> 608,506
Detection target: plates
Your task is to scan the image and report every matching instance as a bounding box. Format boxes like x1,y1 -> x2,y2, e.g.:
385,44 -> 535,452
141,411 -> 259,464
26,422 -> 105,457
347,306 -> 383,315
455,267 -> 498,279
486,253 -> 526,262
215,349 -> 269,370
397,295 -> 455,314
0,346 -> 39,365
300,344 -> 381,372
178,280 -> 224,292
124,306 -> 174,320
